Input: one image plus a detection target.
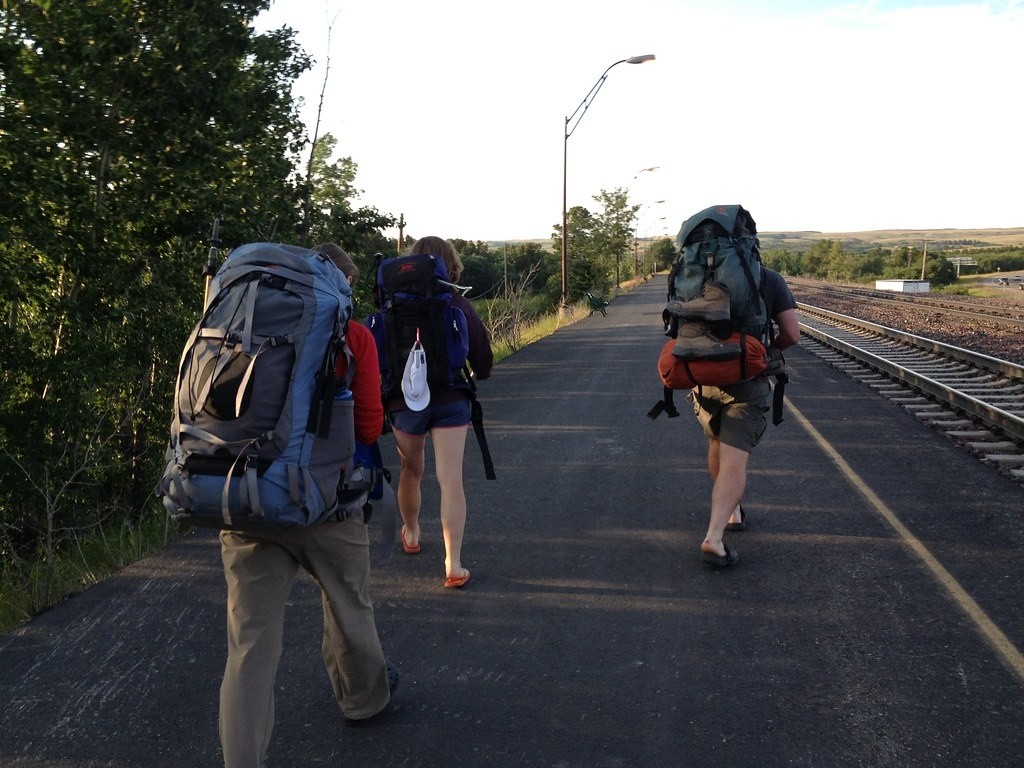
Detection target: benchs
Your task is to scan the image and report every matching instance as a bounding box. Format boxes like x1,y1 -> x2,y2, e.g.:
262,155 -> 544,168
586,291 -> 609,316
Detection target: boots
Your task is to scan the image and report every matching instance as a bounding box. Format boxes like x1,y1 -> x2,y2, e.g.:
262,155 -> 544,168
665,263 -> 733,321
671,316 -> 742,362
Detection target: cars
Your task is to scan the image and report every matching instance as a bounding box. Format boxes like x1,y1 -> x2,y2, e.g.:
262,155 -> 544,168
1014,275 -> 1021,281
999,277 -> 1010,286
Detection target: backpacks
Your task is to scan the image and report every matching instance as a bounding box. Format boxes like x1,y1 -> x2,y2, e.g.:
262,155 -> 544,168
154,242 -> 369,533
664,207 -> 767,338
364,254 -> 470,396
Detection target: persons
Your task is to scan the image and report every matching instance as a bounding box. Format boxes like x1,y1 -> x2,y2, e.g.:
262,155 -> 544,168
217,242 -> 399,768
658,266 -> 800,567
379,235 -> 493,587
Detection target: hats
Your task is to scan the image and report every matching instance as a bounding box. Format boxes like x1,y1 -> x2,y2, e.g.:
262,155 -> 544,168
400,341 -> 430,412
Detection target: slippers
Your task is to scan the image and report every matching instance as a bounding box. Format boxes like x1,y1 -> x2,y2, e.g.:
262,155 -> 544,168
444,568 -> 470,587
726,504 -> 745,531
401,525 -> 421,553
702,543 -> 738,566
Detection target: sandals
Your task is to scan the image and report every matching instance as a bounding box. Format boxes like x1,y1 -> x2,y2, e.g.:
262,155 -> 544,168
344,663 -> 398,728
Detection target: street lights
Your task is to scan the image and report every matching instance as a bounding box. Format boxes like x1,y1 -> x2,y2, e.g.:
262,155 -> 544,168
642,217 -> 666,275
615,164 -> 662,289
635,199 -> 665,276
559,53 -> 656,309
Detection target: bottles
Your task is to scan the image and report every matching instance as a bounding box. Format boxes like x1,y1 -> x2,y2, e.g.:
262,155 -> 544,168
332,374 -> 355,402
